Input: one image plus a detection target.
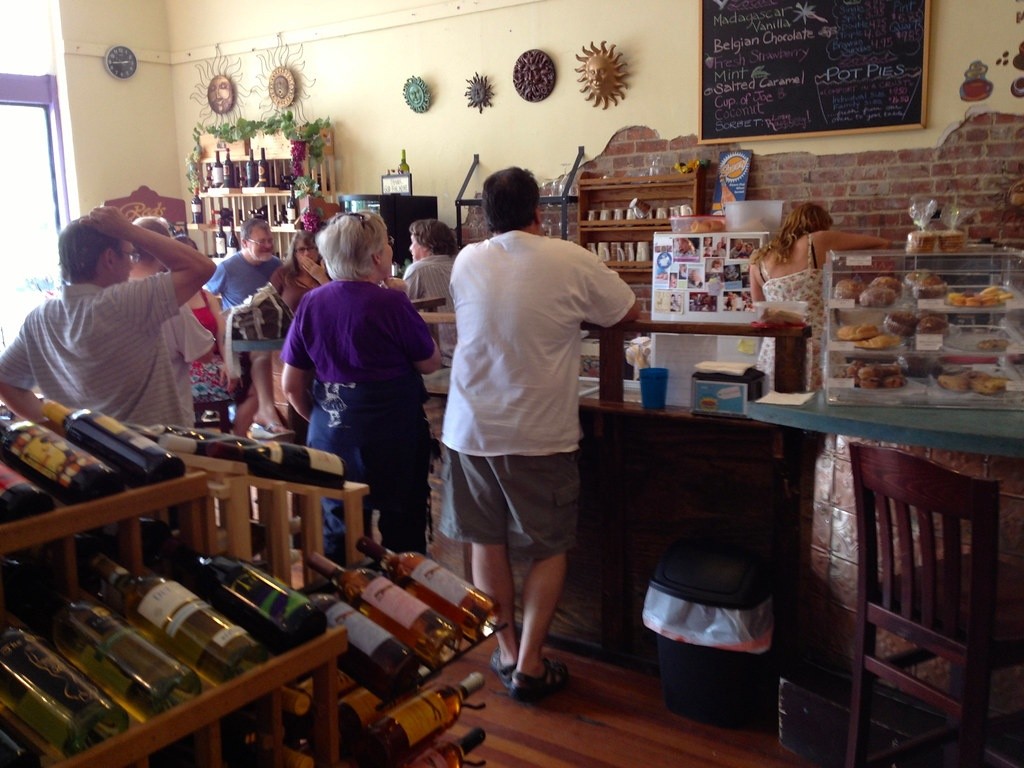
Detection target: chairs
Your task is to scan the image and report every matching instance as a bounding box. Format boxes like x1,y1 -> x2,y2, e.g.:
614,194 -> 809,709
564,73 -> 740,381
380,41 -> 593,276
845,441 -> 1024,768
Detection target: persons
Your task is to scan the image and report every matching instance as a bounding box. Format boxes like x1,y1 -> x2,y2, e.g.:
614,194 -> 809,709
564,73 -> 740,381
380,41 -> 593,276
441,167 -> 641,701
748,204 -> 893,390
130,217 -> 215,428
404,219 -> 458,368
284,210 -> 444,557
670,237 -> 754,312
173,236 -> 294,440
269,232 -> 331,336
205,218 -> 284,310
0,206 -> 216,428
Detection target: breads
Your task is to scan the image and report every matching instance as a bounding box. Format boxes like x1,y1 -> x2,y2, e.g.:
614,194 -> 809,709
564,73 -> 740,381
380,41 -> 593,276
835,271 -> 1013,396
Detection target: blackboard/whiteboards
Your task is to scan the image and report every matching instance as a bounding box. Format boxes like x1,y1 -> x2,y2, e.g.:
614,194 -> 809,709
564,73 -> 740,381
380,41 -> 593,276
698,0 -> 932,145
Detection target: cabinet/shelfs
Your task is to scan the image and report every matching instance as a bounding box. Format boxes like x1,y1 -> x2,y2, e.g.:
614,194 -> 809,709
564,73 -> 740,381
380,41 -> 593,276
187,121 -> 337,299
577,162 -> 707,272
821,250 -> 1024,410
0,450 -> 372,768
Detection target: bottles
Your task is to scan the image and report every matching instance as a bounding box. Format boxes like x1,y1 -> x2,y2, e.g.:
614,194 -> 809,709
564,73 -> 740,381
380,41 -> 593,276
398,149 -> 409,175
191,147 -> 330,258
0,398 -> 506,768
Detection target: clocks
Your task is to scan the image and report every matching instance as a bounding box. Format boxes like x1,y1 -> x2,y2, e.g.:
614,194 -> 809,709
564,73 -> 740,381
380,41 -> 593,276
105,46 -> 138,79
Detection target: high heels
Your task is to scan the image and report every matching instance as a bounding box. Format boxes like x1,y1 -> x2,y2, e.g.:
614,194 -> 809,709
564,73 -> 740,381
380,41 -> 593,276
251,422 -> 295,442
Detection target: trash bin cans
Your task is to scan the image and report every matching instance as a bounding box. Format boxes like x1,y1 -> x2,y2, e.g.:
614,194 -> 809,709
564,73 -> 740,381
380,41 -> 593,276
649,538 -> 787,728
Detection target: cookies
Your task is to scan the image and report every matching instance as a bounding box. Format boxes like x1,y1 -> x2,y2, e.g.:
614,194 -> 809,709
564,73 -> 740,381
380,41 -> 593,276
906,230 -> 965,253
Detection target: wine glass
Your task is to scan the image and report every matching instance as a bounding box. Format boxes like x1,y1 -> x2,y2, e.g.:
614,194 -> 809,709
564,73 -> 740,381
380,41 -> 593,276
536,163 -> 576,236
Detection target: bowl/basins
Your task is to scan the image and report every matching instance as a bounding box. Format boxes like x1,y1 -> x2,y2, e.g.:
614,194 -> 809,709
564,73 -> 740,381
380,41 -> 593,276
724,199 -> 785,232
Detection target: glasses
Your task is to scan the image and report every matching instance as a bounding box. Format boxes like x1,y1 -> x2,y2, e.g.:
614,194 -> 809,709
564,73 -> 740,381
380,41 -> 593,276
110,248 -> 140,263
384,235 -> 394,246
333,213 -> 365,229
247,239 -> 272,245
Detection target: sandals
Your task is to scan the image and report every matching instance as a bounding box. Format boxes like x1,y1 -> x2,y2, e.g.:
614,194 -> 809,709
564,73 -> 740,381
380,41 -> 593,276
509,659 -> 567,699
491,647 -> 515,688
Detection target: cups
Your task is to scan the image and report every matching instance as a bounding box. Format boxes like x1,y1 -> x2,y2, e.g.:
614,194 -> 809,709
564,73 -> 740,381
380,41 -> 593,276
586,241 -> 651,261
639,367 -> 669,412
587,197 -> 693,221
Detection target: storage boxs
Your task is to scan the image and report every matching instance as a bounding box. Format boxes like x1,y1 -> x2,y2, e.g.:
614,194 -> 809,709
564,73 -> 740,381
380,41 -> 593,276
668,214 -> 725,234
725,201 -> 784,232
